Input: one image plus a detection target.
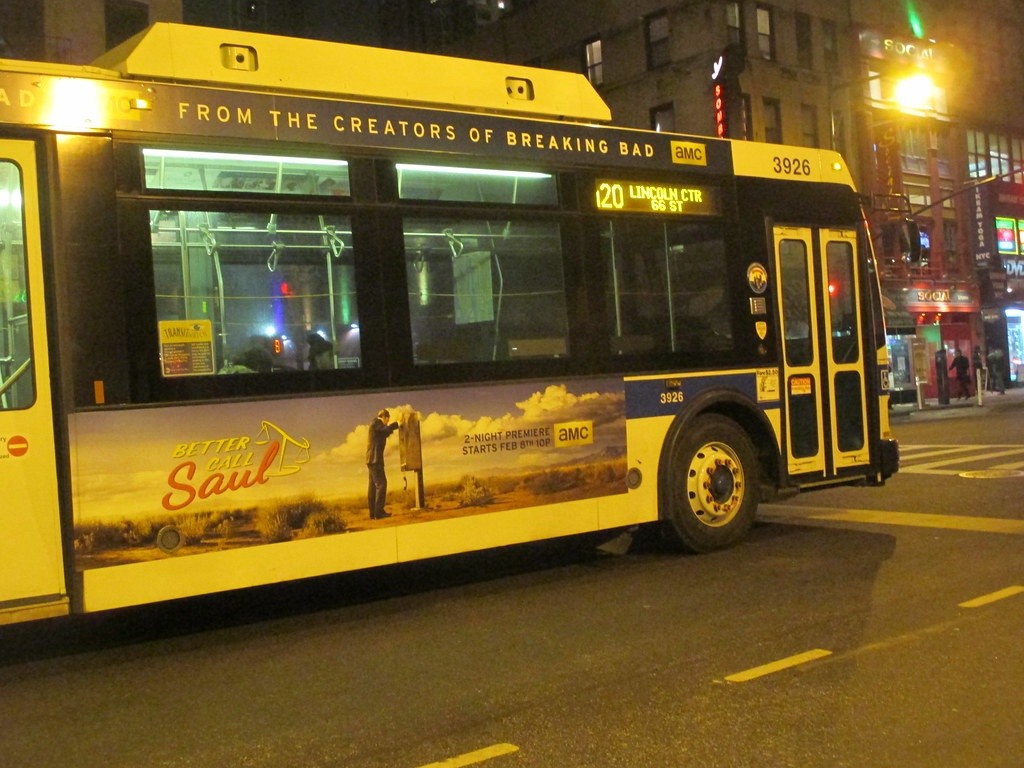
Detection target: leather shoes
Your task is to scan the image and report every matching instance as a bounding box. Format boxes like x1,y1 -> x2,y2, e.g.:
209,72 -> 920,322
370,512 -> 393,520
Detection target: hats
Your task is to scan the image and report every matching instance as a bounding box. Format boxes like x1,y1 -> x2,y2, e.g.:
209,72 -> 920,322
306,333 -> 333,359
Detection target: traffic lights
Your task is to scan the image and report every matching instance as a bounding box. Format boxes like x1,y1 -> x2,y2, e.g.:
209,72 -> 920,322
908,1 -> 940,43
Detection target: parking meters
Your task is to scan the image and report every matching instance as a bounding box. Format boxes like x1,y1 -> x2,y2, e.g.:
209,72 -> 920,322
974,361 -> 983,405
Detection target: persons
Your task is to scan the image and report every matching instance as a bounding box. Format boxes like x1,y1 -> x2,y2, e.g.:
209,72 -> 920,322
218,345 -> 272,374
949,350 -> 970,400
971,344 -> 984,391
365,409 -> 404,519
988,342 -> 1005,395
306,333 -> 336,370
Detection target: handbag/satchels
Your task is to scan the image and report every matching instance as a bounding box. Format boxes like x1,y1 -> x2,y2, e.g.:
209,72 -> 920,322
965,376 -> 970,383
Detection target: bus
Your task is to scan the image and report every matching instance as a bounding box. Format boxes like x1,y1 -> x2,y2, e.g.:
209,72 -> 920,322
1,19 -> 926,629
